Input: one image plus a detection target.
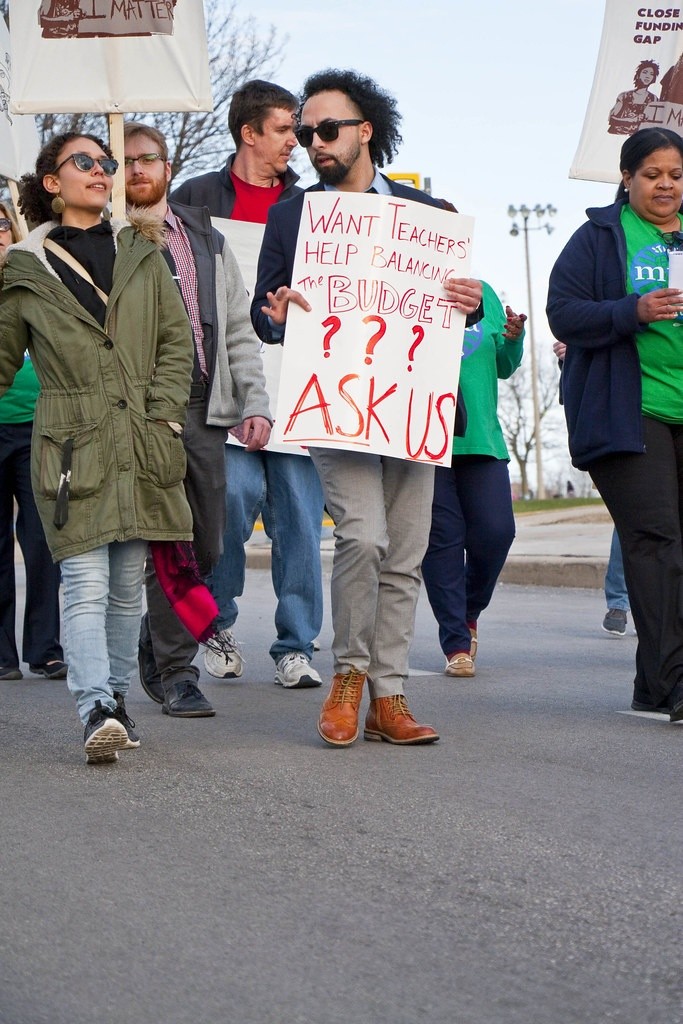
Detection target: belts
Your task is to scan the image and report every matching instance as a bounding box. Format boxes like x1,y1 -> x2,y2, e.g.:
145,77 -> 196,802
190,382 -> 210,400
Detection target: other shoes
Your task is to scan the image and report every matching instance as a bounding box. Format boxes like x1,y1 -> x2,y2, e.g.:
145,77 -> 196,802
29,660 -> 68,679
444,651 -> 476,677
468,621 -> 478,662
0,667 -> 23,680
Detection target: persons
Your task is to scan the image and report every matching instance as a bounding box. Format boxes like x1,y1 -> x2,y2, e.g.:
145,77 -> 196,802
0,133 -> 195,763
250,71 -> 446,749
421,198 -> 527,677
554,341 -> 638,636
546,128 -> 683,725
0,204 -> 68,681
109,122 -> 274,717
566,481 -> 576,498
169,80 -> 324,688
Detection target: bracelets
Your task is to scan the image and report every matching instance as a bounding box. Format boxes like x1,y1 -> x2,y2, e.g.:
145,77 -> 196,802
473,300 -> 481,313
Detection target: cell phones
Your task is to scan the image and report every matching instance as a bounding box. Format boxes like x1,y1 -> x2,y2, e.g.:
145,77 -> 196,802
668,251 -> 683,302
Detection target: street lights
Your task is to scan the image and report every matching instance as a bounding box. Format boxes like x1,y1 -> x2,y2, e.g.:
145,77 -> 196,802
507,203 -> 556,499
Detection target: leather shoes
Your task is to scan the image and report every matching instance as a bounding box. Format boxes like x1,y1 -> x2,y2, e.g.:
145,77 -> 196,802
162,681 -> 216,718
316,672 -> 365,746
138,645 -> 164,703
364,694 -> 440,745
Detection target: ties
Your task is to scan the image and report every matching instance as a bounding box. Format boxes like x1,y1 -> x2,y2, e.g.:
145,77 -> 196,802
159,230 -> 201,380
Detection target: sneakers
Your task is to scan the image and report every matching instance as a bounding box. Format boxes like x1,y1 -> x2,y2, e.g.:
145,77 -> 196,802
113,691 -> 141,749
274,652 -> 322,688
601,609 -> 627,635
84,699 -> 128,764
204,626 -> 246,678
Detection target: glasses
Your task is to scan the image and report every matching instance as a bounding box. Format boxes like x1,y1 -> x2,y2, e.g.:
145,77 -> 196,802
0,218 -> 12,232
294,120 -> 364,147
50,154 -> 119,176
125,154 -> 166,168
